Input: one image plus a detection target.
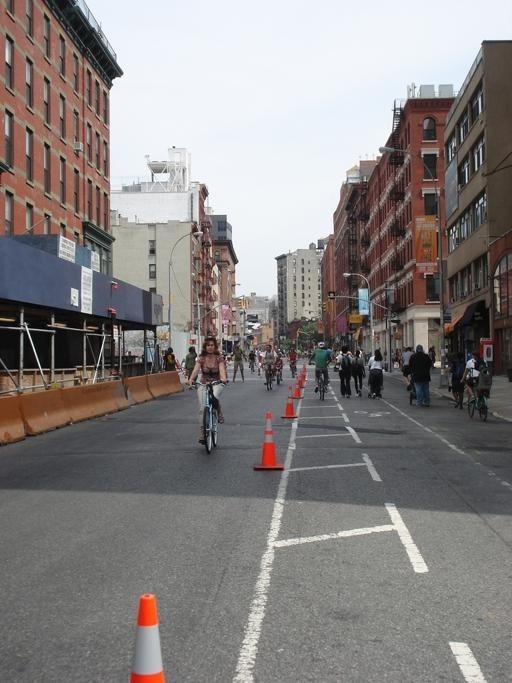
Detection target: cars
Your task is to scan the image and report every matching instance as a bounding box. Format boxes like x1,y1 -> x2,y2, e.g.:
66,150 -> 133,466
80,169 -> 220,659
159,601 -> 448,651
294,362 -> 309,398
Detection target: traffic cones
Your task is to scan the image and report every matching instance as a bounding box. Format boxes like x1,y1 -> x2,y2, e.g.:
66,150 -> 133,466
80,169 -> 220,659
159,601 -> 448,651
129,592 -> 171,682
280,384 -> 299,418
253,410 -> 283,470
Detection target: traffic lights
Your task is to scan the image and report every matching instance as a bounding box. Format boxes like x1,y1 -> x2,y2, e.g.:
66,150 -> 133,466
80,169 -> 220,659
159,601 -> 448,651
239,297 -> 244,309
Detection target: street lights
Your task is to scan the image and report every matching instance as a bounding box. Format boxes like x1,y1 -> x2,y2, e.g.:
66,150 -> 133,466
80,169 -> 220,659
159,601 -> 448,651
343,272 -> 376,356
165,228 -> 205,351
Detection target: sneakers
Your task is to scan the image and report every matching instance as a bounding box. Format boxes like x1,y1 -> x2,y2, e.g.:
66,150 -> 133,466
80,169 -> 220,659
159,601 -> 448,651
341,390 -> 383,399
217,408 -> 224,423
199,426 -> 207,446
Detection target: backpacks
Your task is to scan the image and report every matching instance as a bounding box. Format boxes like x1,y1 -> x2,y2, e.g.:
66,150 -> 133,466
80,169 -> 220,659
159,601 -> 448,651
341,354 -> 351,372
455,365 -> 464,384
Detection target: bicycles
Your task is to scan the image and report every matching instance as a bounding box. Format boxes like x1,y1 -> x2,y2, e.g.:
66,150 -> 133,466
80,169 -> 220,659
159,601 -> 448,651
188,379 -> 225,452
460,377 -> 488,422
308,359 -> 332,400
260,360 -> 297,390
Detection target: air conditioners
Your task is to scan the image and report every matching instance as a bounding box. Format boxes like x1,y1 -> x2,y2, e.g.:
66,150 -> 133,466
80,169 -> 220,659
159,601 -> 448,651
74,142 -> 83,151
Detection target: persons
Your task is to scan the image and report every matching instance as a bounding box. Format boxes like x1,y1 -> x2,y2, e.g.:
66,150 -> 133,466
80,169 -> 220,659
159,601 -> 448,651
308,342 -> 494,410
230,343 -> 299,385
165,347 -> 199,390
186,337 -> 229,443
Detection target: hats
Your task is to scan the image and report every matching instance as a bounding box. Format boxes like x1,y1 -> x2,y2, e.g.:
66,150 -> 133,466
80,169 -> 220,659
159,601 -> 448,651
318,341 -> 326,348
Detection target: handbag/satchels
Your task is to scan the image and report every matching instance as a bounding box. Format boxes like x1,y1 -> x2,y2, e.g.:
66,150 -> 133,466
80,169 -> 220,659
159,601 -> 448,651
478,374 -> 492,390
209,395 -> 219,409
